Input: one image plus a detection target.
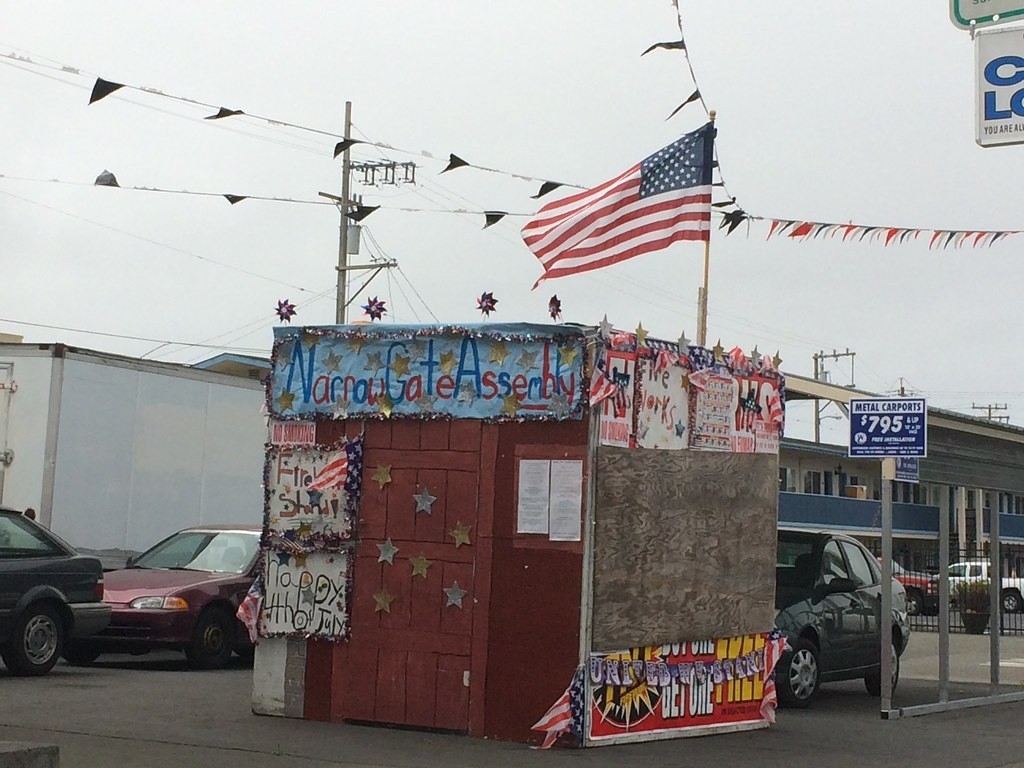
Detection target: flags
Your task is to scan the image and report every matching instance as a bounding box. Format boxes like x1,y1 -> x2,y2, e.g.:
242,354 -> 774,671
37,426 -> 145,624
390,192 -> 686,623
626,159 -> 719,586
236,576 -> 265,643
530,664 -> 585,749
307,439 -> 363,498
521,121 -> 715,291
589,332 -> 785,437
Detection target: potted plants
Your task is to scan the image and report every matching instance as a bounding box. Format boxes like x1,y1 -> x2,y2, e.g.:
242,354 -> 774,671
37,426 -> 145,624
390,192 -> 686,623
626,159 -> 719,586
956,580 -> 991,634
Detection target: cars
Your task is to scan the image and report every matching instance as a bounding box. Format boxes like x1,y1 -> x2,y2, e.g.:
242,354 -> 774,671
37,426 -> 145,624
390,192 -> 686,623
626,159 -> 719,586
876,555 -> 952,616
60,523 -> 263,670
0,504 -> 113,676
771,525 -> 910,708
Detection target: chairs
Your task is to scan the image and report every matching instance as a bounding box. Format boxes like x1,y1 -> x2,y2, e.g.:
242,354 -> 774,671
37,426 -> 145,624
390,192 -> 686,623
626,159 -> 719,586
792,553 -> 823,586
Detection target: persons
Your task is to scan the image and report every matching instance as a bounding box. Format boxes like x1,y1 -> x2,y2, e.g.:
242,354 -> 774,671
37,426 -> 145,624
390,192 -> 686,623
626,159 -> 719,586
24,508 -> 35,520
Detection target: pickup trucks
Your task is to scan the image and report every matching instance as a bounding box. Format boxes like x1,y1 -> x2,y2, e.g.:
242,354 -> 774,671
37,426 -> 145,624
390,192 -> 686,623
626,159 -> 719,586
934,560 -> 1024,614
